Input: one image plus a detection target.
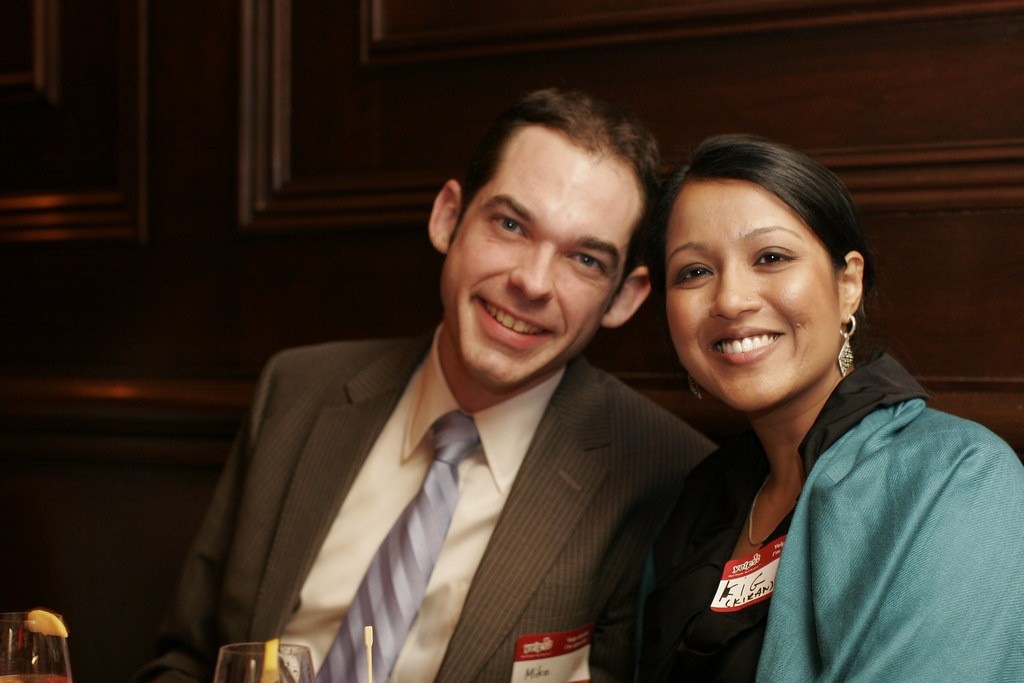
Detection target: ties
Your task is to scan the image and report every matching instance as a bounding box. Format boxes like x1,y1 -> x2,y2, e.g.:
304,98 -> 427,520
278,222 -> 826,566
312,410 -> 480,683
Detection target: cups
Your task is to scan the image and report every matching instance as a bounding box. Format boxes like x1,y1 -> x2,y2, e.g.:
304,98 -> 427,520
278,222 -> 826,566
213,642 -> 316,683
0,612 -> 73,683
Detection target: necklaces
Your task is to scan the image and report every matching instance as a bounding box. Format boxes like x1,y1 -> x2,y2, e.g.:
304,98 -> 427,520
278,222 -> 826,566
746,474 -> 779,548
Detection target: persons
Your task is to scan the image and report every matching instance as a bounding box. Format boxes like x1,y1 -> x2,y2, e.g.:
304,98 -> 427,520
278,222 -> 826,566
620,134 -> 1024,683
142,85 -> 722,681
